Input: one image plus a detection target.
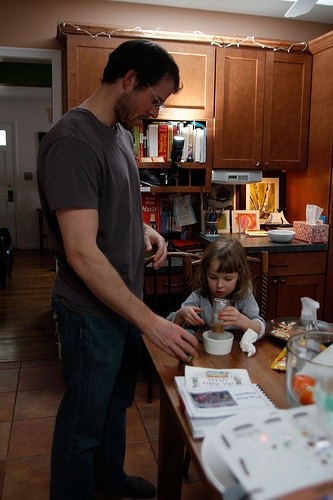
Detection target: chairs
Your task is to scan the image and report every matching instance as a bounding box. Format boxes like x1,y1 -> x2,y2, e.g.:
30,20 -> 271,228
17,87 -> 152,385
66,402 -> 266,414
143,252 -> 203,315
182,251 -> 269,324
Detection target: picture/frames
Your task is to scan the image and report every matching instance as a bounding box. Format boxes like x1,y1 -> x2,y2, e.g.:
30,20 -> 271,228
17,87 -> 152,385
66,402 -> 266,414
231,210 -> 261,233
201,210 -> 231,234
240,171 -> 287,230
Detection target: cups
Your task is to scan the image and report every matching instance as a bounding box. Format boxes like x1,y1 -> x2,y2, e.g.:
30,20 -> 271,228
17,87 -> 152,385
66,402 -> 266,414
283,332 -> 333,408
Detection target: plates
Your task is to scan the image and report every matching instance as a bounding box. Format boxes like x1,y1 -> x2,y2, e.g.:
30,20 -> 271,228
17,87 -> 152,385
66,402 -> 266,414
266,316 -> 333,343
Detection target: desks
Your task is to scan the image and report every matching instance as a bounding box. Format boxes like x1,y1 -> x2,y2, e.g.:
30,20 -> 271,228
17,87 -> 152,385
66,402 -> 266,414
141,330 -> 333,500
194,234 -> 329,323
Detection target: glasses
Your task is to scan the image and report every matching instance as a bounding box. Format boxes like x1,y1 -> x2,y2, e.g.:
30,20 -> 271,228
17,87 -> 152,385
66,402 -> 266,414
144,79 -> 165,111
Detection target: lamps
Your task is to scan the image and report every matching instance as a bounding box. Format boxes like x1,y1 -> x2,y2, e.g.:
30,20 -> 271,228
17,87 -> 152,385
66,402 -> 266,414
170,135 -> 185,179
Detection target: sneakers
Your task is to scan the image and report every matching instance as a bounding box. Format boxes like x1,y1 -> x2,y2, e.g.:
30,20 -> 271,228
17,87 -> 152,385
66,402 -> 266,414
96,476 -> 156,498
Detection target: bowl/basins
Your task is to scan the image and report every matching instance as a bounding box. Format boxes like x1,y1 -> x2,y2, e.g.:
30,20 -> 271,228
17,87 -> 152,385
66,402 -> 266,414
203,330 -> 234,355
267,230 -> 296,242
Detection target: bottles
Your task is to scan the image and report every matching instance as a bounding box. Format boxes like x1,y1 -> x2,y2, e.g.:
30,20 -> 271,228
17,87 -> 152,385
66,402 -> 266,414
211,298 -> 230,333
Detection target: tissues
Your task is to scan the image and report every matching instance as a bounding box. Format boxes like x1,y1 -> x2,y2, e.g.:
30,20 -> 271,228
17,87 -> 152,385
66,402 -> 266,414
291,205 -> 329,245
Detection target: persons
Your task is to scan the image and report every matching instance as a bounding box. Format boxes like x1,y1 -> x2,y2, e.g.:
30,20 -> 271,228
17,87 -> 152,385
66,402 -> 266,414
165,235 -> 266,340
37,39 -> 199,499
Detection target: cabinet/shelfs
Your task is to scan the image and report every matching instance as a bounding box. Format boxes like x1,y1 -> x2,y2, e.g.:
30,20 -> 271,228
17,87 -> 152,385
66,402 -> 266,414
56,22 -> 313,193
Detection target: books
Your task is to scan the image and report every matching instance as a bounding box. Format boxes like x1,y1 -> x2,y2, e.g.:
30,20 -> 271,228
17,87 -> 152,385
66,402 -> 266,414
131,120 -> 206,164
174,364 -> 279,439
